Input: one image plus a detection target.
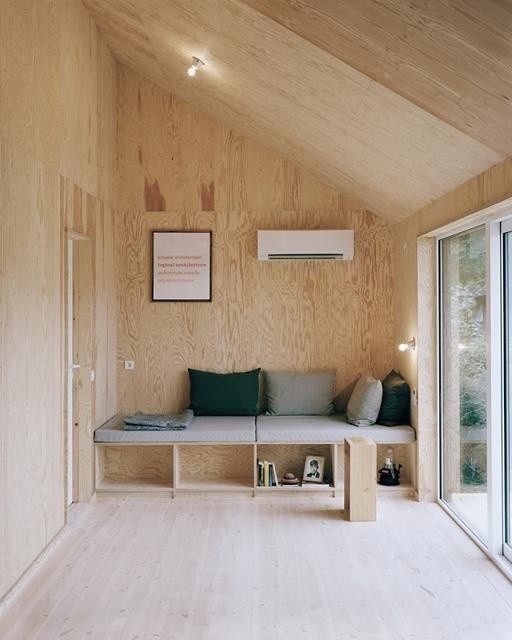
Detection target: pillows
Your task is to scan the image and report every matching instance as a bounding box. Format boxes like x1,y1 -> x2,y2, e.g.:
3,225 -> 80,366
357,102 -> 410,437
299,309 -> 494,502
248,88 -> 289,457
188,368 -> 261,416
376,369 -> 410,427
346,375 -> 383,427
264,369 -> 336,416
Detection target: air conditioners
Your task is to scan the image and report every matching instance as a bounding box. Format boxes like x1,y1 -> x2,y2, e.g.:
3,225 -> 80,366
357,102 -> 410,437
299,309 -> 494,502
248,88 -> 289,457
257,229 -> 354,261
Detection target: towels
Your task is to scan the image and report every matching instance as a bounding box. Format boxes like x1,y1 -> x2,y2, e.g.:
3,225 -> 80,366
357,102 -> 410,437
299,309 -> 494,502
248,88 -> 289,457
124,409 -> 194,430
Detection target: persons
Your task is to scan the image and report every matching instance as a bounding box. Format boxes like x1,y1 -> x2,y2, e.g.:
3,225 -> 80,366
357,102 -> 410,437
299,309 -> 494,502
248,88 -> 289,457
306,460 -> 320,478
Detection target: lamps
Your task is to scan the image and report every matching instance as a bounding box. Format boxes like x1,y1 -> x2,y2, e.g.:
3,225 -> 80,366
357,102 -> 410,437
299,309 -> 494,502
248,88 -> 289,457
399,336 -> 415,351
188,56 -> 205,77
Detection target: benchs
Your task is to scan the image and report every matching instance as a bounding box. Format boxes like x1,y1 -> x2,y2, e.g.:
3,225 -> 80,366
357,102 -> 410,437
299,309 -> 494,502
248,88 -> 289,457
91,417 -> 415,444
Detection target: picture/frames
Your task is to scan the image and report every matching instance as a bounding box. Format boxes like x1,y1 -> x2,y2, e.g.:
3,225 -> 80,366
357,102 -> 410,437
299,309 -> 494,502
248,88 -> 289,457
302,455 -> 325,482
152,231 -> 212,301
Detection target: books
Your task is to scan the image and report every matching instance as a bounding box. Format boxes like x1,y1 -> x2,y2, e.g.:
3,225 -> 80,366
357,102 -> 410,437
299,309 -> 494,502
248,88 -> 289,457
258,461 -> 279,487
